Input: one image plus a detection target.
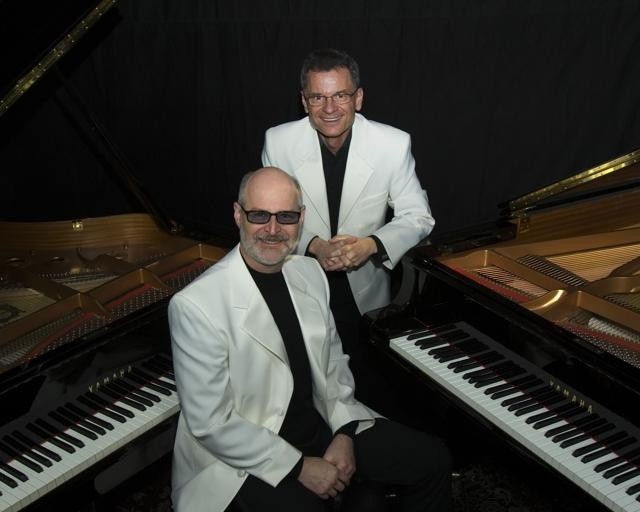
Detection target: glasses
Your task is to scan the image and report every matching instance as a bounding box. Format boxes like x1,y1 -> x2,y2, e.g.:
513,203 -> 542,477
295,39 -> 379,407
303,87 -> 359,106
240,205 -> 301,225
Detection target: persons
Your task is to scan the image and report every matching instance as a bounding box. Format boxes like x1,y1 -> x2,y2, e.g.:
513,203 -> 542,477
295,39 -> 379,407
165,167 -> 455,511
261,47 -> 438,383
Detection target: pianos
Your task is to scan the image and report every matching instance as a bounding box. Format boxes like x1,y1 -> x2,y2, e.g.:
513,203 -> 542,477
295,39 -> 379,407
361,148 -> 638,507
0,1 -> 231,507
